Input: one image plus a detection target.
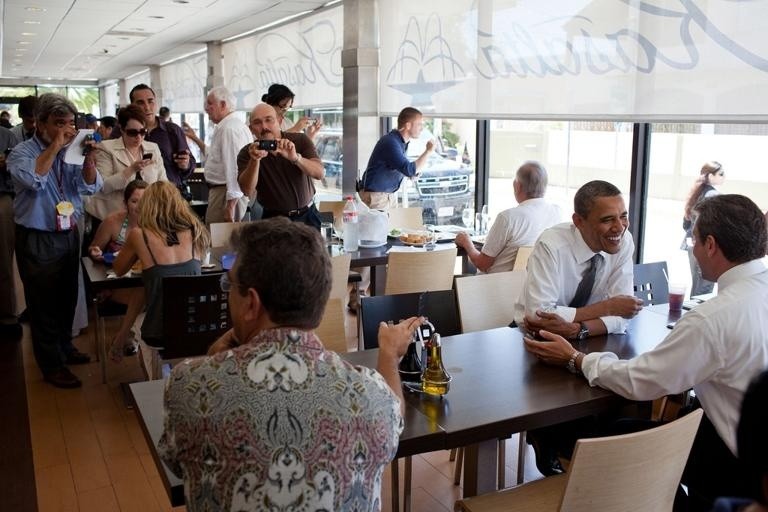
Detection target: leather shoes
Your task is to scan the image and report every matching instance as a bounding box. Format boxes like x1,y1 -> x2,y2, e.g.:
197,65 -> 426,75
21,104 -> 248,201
44,368 -> 82,388
532,443 -> 566,477
61,347 -> 92,364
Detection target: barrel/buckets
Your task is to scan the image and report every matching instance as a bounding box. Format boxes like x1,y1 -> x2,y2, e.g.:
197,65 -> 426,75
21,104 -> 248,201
359,207 -> 391,250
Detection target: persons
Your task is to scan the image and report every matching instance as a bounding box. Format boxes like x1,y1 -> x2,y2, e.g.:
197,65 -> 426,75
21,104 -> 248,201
348,107 -> 435,314
679,160 -> 727,300
713,369 -> 768,512
455,160 -> 563,275
154,216 -> 426,512
2,80 -> 325,390
522,194 -> 768,512
508,180 -> 645,479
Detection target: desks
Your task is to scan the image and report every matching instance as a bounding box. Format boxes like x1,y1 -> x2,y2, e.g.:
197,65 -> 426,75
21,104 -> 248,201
646,297 -> 693,409
348,325 -> 618,498
129,324 -> 445,508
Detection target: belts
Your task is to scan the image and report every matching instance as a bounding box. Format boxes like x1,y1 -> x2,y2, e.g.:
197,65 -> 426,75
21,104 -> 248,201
287,200 -> 313,217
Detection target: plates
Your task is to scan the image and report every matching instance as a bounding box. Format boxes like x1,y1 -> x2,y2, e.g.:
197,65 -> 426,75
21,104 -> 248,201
434,224 -> 486,245
387,232 -> 422,246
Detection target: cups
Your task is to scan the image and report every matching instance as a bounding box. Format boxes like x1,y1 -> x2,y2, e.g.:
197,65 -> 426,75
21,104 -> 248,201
667,285 -> 684,311
320,221 -> 333,241
424,224 -> 436,250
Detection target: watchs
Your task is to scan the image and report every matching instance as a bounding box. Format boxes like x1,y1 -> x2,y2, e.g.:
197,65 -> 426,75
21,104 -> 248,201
566,351 -> 583,375
577,322 -> 591,342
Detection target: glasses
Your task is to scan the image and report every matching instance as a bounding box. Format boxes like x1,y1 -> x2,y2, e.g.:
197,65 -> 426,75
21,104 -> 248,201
687,238 -> 706,246
279,105 -> 291,111
220,273 -> 242,292
122,128 -> 148,137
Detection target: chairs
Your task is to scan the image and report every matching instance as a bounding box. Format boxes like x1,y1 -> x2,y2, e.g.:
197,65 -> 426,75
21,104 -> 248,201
360,289 -> 461,510
452,408 -> 703,512
634,261 -> 671,307
726,373 -> 768,510
84,164 -> 536,381
456,270 -> 529,488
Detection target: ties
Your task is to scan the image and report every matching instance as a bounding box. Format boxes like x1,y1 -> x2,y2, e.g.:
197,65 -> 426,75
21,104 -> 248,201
569,254 -> 604,308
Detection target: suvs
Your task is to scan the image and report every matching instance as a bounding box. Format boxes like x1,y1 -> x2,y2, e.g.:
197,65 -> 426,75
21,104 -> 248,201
312,126 -> 474,226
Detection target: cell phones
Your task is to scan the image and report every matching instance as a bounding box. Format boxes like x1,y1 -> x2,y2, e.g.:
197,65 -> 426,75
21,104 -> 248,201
143,153 -> 152,160
175,150 -> 186,159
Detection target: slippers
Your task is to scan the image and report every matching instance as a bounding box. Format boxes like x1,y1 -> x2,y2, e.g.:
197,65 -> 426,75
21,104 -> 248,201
108,331 -> 138,364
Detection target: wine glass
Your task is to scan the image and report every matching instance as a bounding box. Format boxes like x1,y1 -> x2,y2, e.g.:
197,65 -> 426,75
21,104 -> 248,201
462,204 -> 494,234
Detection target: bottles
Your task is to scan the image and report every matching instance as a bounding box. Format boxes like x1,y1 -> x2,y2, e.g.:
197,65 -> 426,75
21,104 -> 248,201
343,197 -> 357,253
396,329 -> 452,394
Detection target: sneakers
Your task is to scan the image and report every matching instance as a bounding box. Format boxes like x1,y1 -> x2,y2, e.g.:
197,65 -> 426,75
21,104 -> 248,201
98,298 -> 129,316
349,289 -> 366,310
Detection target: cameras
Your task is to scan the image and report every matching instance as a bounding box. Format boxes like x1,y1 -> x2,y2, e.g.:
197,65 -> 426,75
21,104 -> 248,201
258,140 -> 277,150
306,119 -> 317,127
